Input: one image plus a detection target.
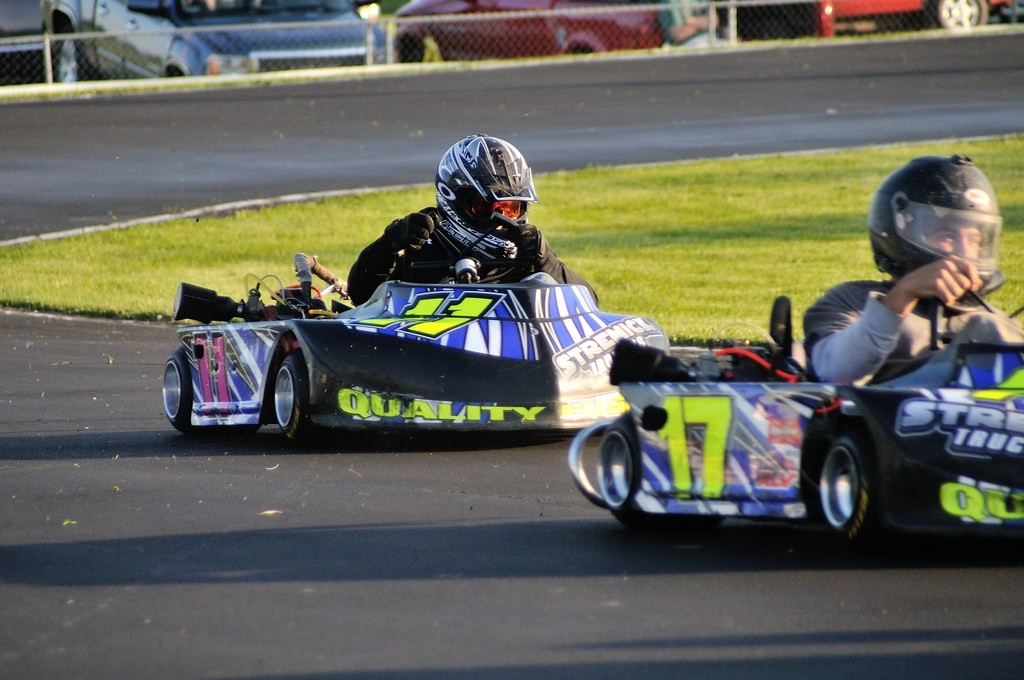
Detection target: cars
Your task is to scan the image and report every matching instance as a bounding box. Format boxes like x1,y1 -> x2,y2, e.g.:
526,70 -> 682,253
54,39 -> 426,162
0,0 -> 1024,85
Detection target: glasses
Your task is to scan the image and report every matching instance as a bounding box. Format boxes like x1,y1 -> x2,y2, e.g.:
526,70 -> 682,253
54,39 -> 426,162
488,200 -> 525,221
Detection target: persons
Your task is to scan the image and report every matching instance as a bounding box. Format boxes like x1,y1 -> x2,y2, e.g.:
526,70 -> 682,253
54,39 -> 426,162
348,132 -> 599,310
802,154 -> 1024,390
659,0 -> 719,48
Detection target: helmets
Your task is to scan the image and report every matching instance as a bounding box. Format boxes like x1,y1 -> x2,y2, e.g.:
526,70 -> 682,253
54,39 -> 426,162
435,133 -> 539,262
867,154 -> 1003,311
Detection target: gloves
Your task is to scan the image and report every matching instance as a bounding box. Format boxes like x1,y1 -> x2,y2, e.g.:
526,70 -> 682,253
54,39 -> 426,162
384,213 -> 434,257
502,224 -> 541,266
